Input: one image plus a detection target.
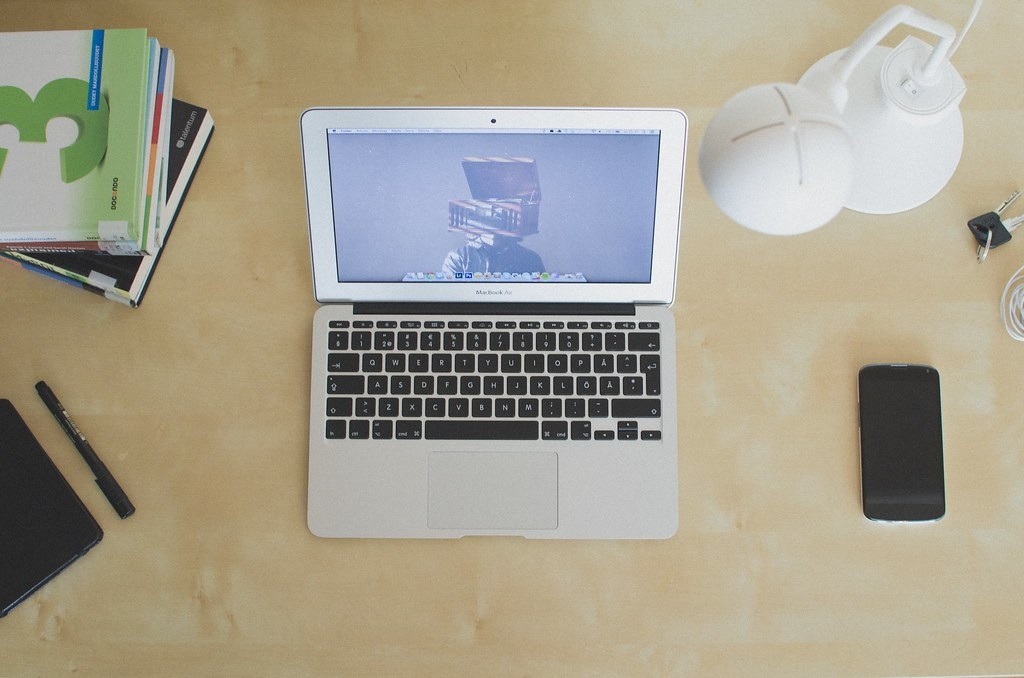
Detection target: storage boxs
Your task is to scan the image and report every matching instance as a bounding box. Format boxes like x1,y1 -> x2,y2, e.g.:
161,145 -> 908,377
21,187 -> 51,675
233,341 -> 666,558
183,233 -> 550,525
449,157 -> 540,236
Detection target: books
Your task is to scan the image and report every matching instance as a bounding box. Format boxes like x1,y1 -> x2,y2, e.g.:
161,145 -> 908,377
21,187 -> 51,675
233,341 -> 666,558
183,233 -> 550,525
0,28 -> 214,308
0,400 -> 104,617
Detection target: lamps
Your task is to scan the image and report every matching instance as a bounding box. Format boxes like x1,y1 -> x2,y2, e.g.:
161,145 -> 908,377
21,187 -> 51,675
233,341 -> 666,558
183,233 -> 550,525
696,2 -> 968,236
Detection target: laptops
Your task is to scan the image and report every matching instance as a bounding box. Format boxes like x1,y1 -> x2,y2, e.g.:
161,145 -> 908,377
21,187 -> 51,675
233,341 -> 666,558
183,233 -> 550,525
300,107 -> 689,540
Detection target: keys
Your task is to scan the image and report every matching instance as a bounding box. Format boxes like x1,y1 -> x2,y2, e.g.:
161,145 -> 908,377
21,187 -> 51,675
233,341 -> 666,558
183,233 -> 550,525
968,190 -> 1024,262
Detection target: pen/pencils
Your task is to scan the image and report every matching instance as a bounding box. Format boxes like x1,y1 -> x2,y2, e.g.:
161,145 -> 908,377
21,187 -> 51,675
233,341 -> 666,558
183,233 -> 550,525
34,380 -> 135,521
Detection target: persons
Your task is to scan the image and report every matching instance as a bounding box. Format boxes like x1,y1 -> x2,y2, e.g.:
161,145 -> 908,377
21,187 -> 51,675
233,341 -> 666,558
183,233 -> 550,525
443,235 -> 545,273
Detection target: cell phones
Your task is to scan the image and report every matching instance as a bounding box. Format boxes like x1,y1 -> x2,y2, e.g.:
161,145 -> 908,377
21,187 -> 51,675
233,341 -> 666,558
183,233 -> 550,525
856,364 -> 946,523
